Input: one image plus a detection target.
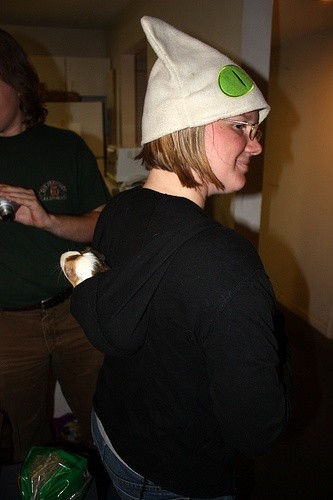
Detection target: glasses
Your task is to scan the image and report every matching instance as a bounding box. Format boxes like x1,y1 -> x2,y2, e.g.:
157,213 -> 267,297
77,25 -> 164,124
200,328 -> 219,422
219,118 -> 265,143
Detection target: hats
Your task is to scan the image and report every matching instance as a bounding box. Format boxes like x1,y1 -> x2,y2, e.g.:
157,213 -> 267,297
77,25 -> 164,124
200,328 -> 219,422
139,14 -> 273,143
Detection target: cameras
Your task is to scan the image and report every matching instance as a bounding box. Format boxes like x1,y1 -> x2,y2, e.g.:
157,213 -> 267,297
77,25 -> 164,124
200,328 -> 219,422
0,197 -> 20,223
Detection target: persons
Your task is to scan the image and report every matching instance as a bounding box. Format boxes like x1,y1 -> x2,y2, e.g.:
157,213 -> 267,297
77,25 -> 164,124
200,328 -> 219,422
0,47 -> 114,310
48,18 -> 333,499
